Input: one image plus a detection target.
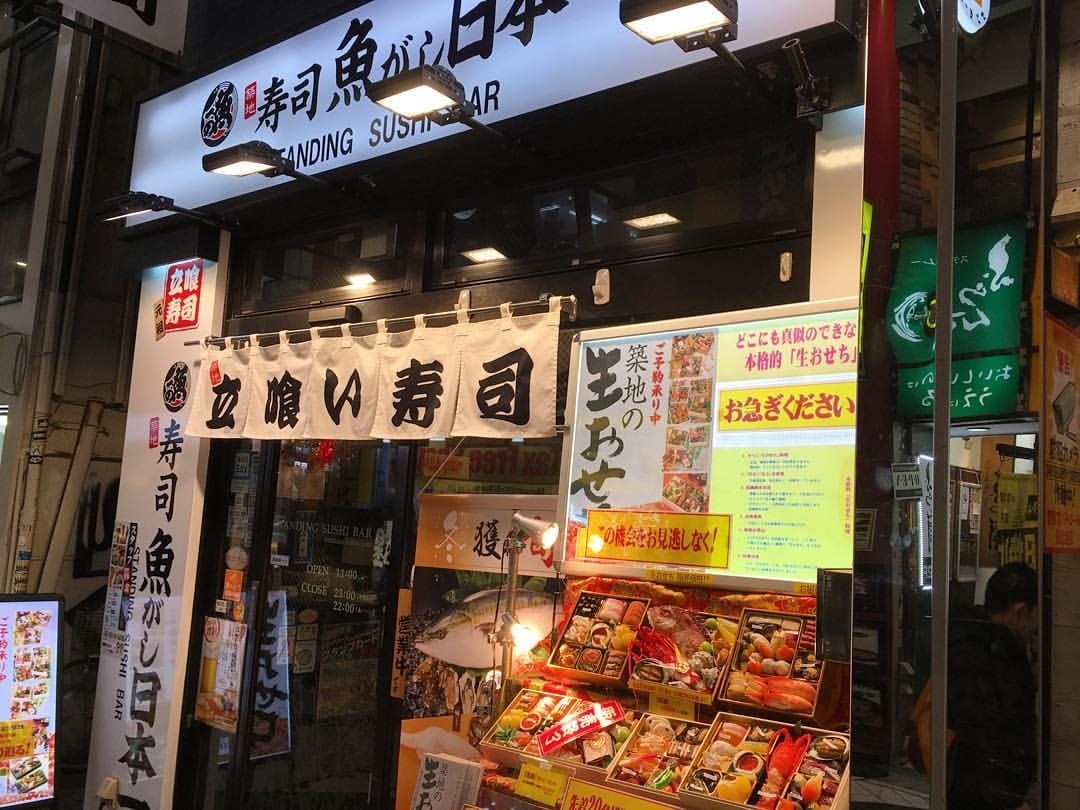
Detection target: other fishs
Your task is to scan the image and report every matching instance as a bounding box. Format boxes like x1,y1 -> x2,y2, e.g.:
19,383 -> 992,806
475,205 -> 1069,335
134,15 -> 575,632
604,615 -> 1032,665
647,605 -> 712,652
414,588 -> 562,670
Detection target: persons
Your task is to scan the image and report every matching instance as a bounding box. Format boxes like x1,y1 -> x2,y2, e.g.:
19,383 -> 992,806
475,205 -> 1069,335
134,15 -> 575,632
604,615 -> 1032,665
946,559 -> 1042,810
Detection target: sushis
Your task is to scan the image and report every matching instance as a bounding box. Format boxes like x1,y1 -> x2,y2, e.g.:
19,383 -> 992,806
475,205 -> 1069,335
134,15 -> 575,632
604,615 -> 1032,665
490,595 -> 850,810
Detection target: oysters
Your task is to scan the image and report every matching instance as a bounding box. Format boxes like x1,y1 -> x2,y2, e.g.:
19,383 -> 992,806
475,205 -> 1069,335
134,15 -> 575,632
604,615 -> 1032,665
409,657 -> 497,717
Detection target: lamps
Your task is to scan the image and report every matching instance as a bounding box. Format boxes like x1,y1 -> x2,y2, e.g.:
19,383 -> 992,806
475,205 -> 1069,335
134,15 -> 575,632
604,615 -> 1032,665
102,191 -> 221,229
620,0 -> 779,115
493,512 -> 559,716
368,65 -> 505,142
202,141 -> 330,189
